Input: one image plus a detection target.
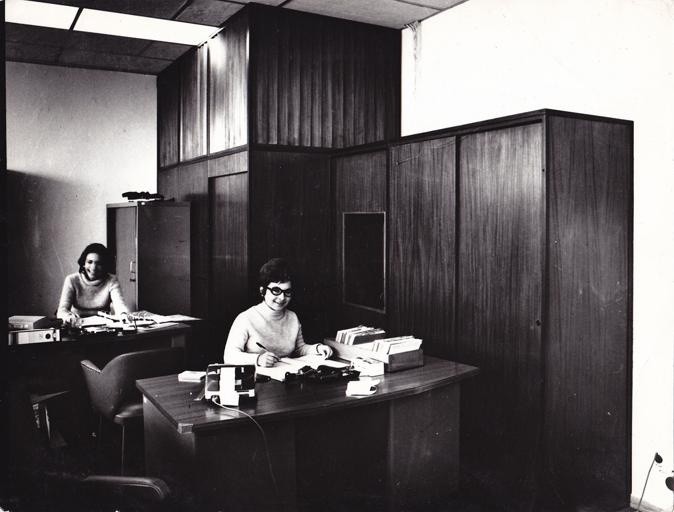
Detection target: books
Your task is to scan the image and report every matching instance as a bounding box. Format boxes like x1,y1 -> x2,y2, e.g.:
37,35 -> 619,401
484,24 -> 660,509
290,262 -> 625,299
335,324 -> 423,356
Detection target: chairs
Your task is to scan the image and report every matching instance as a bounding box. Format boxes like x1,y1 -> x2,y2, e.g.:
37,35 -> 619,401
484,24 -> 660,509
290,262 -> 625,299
82,342 -> 183,475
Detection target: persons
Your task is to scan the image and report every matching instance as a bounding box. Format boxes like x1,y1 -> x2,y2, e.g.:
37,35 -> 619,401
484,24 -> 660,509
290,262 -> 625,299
222,257 -> 333,368
56,243 -> 133,325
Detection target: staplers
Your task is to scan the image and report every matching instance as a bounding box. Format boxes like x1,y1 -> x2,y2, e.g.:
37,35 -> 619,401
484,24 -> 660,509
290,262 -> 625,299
189,380 -> 205,402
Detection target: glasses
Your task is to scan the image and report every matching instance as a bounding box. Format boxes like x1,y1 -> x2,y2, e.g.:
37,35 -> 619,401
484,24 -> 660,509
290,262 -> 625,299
267,286 -> 293,297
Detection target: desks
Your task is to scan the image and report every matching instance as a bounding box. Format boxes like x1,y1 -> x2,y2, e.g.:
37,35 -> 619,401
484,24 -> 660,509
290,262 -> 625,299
134,356 -> 480,512
2,322 -> 193,360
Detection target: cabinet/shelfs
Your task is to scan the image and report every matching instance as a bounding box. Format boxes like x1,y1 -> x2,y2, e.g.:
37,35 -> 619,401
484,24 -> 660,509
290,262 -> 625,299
328,110 -> 630,510
155,2 -> 404,346
107,200 -> 194,316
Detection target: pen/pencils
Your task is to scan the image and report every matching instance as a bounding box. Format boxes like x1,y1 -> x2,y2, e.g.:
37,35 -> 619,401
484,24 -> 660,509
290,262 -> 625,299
256,342 -> 278,361
69,311 -> 85,322
